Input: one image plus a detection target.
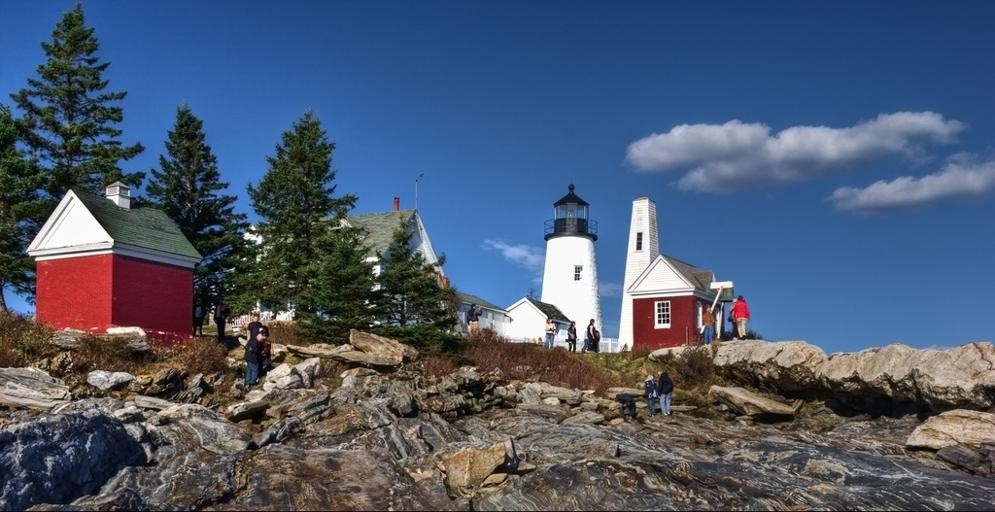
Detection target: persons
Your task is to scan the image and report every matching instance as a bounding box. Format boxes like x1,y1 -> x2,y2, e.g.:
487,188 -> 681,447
243,331 -> 266,385
465,303 -> 482,336
246,312 -> 269,367
192,304 -> 206,337
702,307 -> 716,344
734,295 -> 750,339
615,394 -> 636,420
657,369 -> 673,416
536,318 -> 599,353
644,374 -> 658,417
214,302 -> 230,336
